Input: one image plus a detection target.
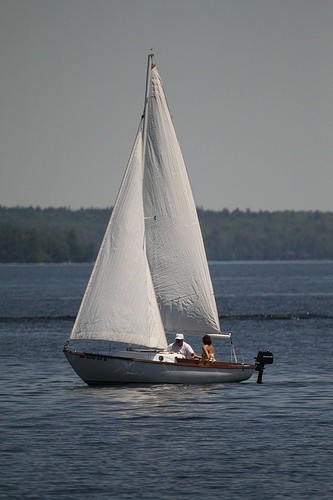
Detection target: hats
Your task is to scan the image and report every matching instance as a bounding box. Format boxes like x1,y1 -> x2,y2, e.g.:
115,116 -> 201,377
175,333 -> 184,340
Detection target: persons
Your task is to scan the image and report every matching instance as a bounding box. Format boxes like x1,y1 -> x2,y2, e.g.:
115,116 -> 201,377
191,335 -> 216,362
165,334 -> 197,359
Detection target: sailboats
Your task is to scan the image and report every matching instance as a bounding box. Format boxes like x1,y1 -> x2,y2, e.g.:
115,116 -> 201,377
61,47 -> 259,386
